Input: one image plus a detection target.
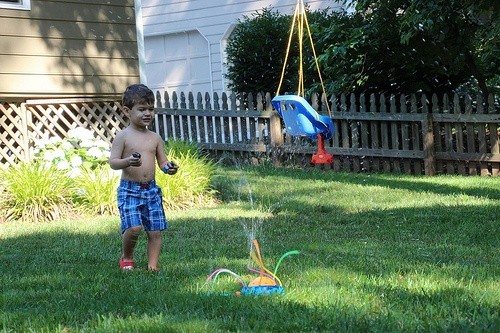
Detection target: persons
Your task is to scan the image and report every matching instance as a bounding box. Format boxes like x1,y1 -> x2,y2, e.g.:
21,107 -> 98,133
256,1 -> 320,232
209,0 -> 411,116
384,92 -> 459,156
109,84 -> 179,273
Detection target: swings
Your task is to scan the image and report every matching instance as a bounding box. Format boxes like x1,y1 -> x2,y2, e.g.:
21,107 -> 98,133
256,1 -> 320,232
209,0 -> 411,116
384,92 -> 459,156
269,1 -> 336,143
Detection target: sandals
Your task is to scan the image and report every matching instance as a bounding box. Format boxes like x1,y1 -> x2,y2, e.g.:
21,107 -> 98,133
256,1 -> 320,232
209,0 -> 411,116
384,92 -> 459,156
119,256 -> 134,269
